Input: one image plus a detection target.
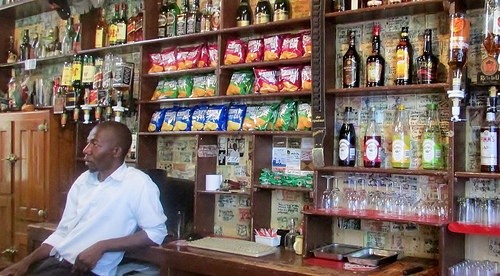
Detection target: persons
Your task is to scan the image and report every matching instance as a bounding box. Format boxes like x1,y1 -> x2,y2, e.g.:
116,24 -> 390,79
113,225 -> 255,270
1,120 -> 168,276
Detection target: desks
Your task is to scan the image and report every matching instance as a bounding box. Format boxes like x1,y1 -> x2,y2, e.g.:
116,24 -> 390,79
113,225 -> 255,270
26,222 -> 439,276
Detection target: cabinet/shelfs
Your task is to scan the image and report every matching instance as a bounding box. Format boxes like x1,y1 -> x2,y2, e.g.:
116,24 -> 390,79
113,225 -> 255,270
0,0 -> 500,276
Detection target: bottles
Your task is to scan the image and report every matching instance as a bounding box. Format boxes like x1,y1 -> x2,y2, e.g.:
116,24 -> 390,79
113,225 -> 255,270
416,29 -> 438,84
338,107 -> 357,167
9,54 -> 129,126
479,87 -> 499,171
284,218 -> 298,250
395,27 -> 413,86
254,0 -> 271,25
483,0 -> 500,62
367,35 -> 385,87
158,0 -> 214,39
421,104 -> 443,169
94,3 -> 143,49
273,0 -> 289,22
342,31 -> 360,87
363,108 -> 382,168
333,0 -> 402,13
449,14 -> 470,90
20,14 -> 83,62
235,0 -> 251,27
392,105 -> 411,168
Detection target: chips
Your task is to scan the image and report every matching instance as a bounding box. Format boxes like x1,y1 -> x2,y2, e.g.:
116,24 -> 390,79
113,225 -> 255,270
148,44 -> 312,131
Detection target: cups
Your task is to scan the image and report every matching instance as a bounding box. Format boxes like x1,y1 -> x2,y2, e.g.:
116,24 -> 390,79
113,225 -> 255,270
448,259 -> 499,276
206,174 -> 221,192
456,198 -> 500,227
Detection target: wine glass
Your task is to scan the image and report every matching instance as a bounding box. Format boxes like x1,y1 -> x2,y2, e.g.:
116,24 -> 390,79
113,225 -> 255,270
321,176 -> 447,221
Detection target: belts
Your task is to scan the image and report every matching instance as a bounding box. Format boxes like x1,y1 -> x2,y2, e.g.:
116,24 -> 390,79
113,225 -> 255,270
50,247 -> 74,269
